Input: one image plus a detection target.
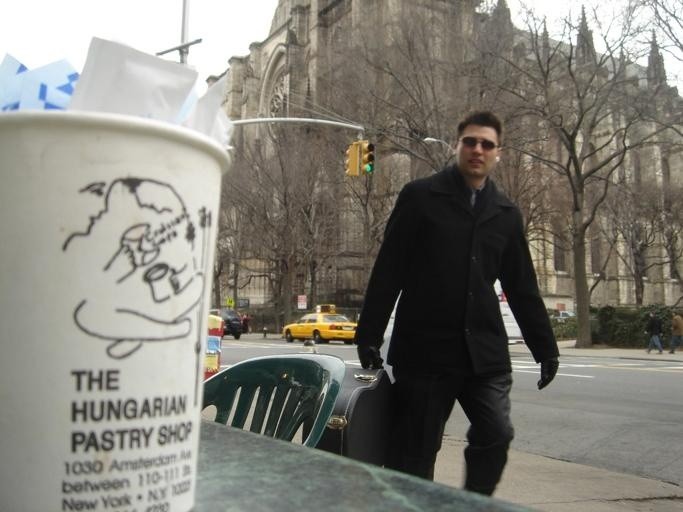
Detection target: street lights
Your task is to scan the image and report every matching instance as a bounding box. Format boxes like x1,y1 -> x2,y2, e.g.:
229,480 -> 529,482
423,137 -> 456,155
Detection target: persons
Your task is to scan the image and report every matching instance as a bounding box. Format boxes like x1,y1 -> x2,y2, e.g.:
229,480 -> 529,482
243,312 -> 254,333
669,312 -> 683,353
644,311 -> 663,354
354,113 -> 560,496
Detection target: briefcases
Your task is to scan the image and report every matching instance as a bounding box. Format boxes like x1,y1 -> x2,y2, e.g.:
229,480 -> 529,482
302,362 -> 394,467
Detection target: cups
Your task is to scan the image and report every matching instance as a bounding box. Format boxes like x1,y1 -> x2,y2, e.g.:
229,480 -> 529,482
1,111 -> 232,512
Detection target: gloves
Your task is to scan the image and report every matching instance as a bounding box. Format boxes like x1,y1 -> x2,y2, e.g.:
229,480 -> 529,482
537,356 -> 559,390
358,343 -> 384,369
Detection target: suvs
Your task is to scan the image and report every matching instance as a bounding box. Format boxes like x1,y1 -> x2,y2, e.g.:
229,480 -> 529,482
208,309 -> 244,339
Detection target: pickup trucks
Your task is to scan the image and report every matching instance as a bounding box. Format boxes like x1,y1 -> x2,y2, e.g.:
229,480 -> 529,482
550,310 -> 576,323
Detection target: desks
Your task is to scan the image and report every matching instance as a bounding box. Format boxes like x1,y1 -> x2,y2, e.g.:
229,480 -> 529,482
184,417 -> 535,512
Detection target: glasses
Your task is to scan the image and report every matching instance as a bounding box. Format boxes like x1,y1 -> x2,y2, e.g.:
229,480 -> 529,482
459,138 -> 500,150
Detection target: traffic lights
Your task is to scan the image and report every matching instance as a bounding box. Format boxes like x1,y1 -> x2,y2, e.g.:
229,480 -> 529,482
345,140 -> 374,177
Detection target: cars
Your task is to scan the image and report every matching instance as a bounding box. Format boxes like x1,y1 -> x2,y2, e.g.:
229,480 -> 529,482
282,312 -> 358,344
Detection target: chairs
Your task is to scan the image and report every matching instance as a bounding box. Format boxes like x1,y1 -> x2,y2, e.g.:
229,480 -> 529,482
186,350 -> 348,453
